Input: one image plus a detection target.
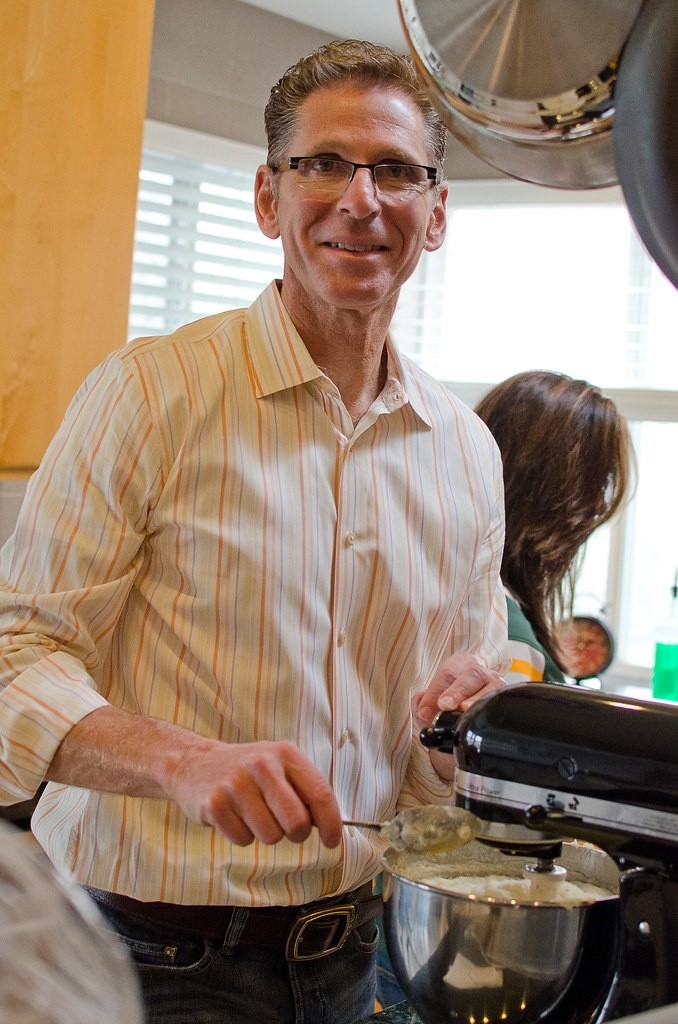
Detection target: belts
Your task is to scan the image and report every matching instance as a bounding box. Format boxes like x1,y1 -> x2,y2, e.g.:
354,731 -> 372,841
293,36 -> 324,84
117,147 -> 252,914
77,878 -> 386,964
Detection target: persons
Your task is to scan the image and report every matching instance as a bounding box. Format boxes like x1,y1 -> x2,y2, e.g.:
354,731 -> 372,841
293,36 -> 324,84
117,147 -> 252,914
0,41 -> 513,1024
373,370 -> 632,1009
0,822 -> 145,1024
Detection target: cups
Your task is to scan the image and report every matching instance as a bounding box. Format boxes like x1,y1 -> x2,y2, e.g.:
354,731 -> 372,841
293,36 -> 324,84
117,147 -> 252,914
649,640 -> 678,703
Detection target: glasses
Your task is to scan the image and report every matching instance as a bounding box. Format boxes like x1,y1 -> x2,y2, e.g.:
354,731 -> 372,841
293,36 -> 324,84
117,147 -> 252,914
268,156 -> 441,196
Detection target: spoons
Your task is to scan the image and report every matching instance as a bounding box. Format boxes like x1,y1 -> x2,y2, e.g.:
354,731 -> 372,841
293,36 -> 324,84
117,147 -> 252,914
342,804 -> 481,854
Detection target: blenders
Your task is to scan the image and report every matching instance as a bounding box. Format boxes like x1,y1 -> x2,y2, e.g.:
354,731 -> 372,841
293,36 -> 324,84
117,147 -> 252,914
376,800 -> 644,1024
